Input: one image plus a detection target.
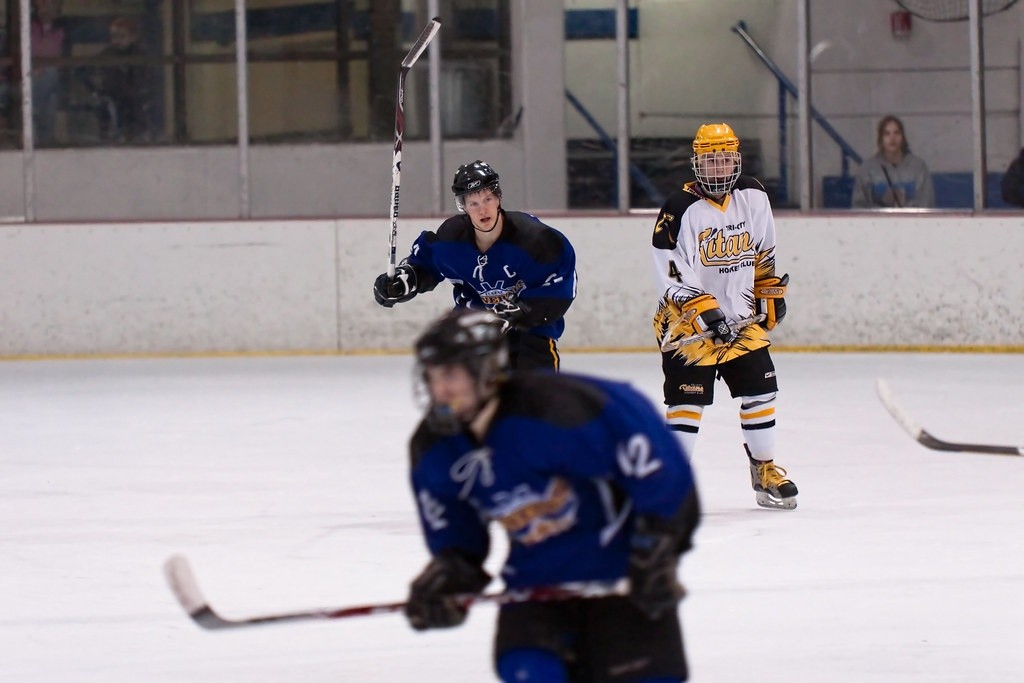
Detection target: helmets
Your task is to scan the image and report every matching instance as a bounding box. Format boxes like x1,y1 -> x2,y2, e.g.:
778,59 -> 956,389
452,159 -> 499,211
690,123 -> 742,196
413,311 -> 511,432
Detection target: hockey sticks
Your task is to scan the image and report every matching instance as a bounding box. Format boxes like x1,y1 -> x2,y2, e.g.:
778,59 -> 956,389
158,553 -> 636,635
660,307 -> 768,355
382,13 -> 443,309
872,377 -> 1024,457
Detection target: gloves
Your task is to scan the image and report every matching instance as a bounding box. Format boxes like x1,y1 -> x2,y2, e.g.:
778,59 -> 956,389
491,295 -> 532,327
680,294 -> 734,347
751,273 -> 789,332
629,524 -> 688,617
374,263 -> 419,308
407,555 -> 491,628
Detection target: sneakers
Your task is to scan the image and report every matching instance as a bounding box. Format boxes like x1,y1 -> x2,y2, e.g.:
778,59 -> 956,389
744,441 -> 798,510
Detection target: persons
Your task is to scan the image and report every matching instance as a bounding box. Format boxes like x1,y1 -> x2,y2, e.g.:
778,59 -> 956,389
374,161 -> 578,374
852,115 -> 934,207
1002,147 -> 1024,208
652,121 -> 799,511
0,0 -> 152,145
402,311 -> 704,683
